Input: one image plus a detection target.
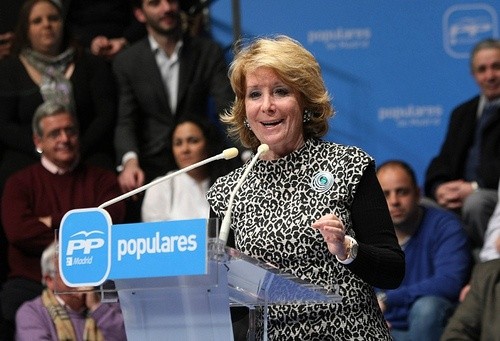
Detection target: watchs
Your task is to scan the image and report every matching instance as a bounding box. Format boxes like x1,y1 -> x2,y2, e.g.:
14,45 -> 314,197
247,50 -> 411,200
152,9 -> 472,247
336,235 -> 359,264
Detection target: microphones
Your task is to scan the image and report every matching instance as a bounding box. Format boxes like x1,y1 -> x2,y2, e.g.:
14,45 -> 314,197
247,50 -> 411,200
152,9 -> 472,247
219,144 -> 269,243
98,147 -> 238,209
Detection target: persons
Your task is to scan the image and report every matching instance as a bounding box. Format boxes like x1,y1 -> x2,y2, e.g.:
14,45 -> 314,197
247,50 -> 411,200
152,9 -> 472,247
0,101 -> 122,341
15,240 -> 127,341
60,0 -> 146,61
111,0 -> 248,224
0,0 -> 114,170
376,160 -> 476,341
441,230 -> 500,341
206,35 -> 405,341
423,38 -> 500,249
140,118 -> 216,223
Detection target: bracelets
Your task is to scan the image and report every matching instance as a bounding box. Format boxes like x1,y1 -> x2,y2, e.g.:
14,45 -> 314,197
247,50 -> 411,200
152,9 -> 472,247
470,181 -> 479,191
